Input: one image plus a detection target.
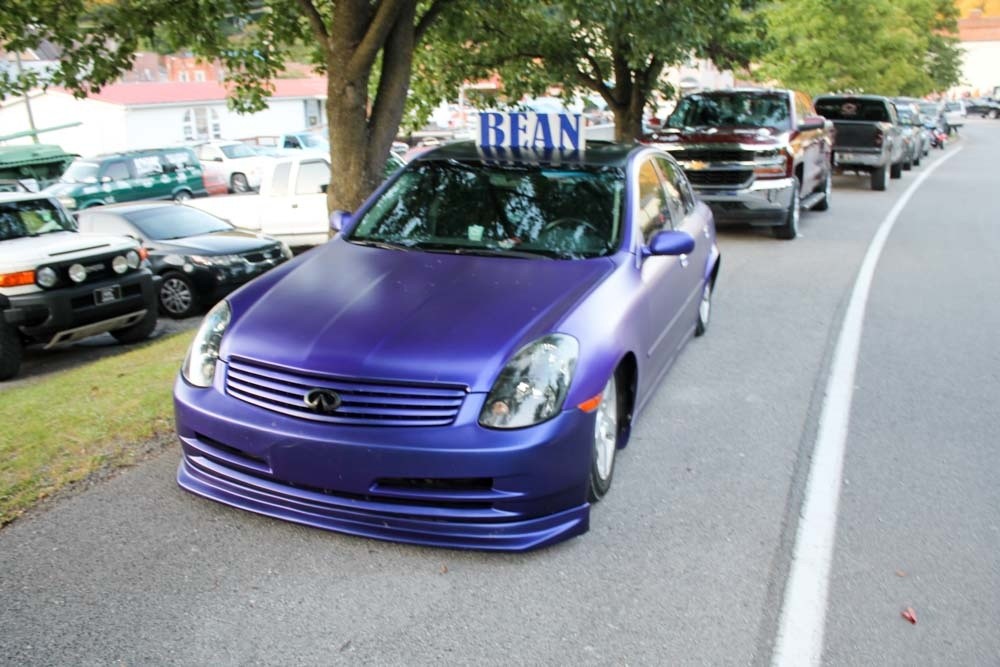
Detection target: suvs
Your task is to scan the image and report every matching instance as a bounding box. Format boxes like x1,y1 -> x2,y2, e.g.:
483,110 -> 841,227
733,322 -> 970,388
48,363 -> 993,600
0,192 -> 164,381
643,87 -> 838,237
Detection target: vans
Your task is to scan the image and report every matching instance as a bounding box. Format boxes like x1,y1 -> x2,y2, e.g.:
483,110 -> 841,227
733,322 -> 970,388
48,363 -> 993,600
36,146 -> 208,220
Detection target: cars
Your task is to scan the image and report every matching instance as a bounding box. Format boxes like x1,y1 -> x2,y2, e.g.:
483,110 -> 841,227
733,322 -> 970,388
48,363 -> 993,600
0,177 -> 38,193
79,201 -> 297,320
889,94 -> 1000,168
170,111 -> 721,556
192,125 -> 332,196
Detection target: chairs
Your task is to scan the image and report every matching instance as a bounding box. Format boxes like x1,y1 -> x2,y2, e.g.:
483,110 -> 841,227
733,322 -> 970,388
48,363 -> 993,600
698,106 -> 718,122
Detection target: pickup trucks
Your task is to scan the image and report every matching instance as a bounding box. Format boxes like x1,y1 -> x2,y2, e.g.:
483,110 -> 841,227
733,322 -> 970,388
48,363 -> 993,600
811,95 -> 907,192
